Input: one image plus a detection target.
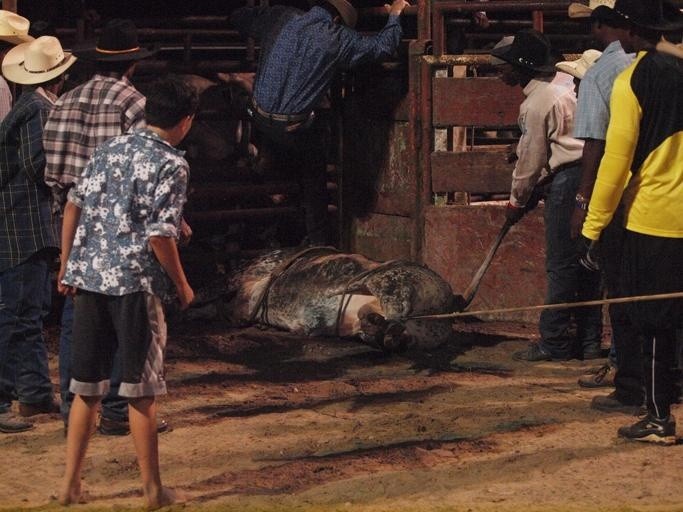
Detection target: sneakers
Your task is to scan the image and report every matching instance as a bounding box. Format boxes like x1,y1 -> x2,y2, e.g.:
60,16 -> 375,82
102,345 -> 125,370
18,398 -> 61,417
511,338 -> 572,363
590,390 -> 648,416
617,413 -> 678,445
98,415 -> 170,437
0,406 -> 36,433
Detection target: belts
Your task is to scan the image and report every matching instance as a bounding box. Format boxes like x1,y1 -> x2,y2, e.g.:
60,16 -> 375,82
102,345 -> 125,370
250,96 -> 308,123
556,159 -> 583,170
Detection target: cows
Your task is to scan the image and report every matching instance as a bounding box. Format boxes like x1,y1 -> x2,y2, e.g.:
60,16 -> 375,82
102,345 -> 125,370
190,181 -> 546,352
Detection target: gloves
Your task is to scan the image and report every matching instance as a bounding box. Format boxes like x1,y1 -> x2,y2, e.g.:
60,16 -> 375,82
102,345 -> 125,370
574,234 -> 601,274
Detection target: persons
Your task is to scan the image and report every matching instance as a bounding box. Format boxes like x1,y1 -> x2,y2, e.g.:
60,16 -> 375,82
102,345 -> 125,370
0,6 -> 198,435
59,79 -> 198,510
236,0 -> 414,243
568,2 -> 646,415
490,28 -> 618,388
576,0 -> 683,444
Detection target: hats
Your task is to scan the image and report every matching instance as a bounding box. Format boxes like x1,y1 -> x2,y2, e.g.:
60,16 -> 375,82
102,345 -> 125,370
0,35 -> 78,85
554,48 -> 602,80
627,0 -> 683,32
490,27 -> 557,76
569,4 -> 593,18
0,8 -> 35,44
94,16 -> 150,61
327,0 -> 359,30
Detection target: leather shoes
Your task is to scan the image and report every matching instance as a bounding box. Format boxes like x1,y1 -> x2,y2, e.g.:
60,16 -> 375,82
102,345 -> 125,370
577,364 -> 619,389
